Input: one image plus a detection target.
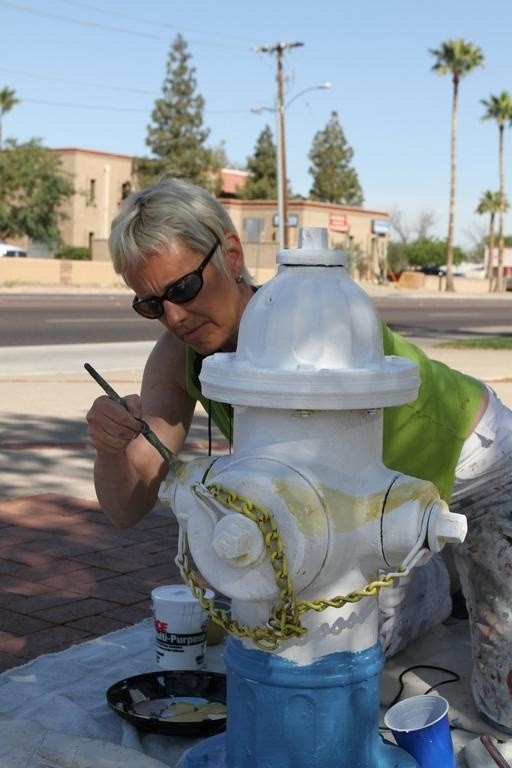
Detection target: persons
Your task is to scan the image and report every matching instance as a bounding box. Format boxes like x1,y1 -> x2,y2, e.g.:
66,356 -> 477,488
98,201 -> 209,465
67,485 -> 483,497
83,175 -> 512,734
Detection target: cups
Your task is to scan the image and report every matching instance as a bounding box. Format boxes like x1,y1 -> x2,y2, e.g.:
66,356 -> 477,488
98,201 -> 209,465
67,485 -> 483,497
386,696 -> 457,767
150,585 -> 215,672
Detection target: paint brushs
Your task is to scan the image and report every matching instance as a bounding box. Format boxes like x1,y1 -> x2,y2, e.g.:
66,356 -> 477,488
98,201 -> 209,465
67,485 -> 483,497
83,363 -> 186,476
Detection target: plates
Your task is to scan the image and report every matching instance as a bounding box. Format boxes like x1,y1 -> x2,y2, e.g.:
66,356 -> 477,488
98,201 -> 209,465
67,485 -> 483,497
107,669 -> 227,737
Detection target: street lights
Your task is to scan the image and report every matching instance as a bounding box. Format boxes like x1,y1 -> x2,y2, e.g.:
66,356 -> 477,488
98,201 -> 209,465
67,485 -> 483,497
251,80 -> 333,248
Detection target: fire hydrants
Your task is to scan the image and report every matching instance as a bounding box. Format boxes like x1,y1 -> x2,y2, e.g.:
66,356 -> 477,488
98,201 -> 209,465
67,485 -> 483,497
158,226 -> 468,762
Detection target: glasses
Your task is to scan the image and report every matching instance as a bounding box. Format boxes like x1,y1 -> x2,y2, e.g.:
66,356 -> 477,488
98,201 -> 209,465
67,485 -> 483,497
132,238 -> 222,320
